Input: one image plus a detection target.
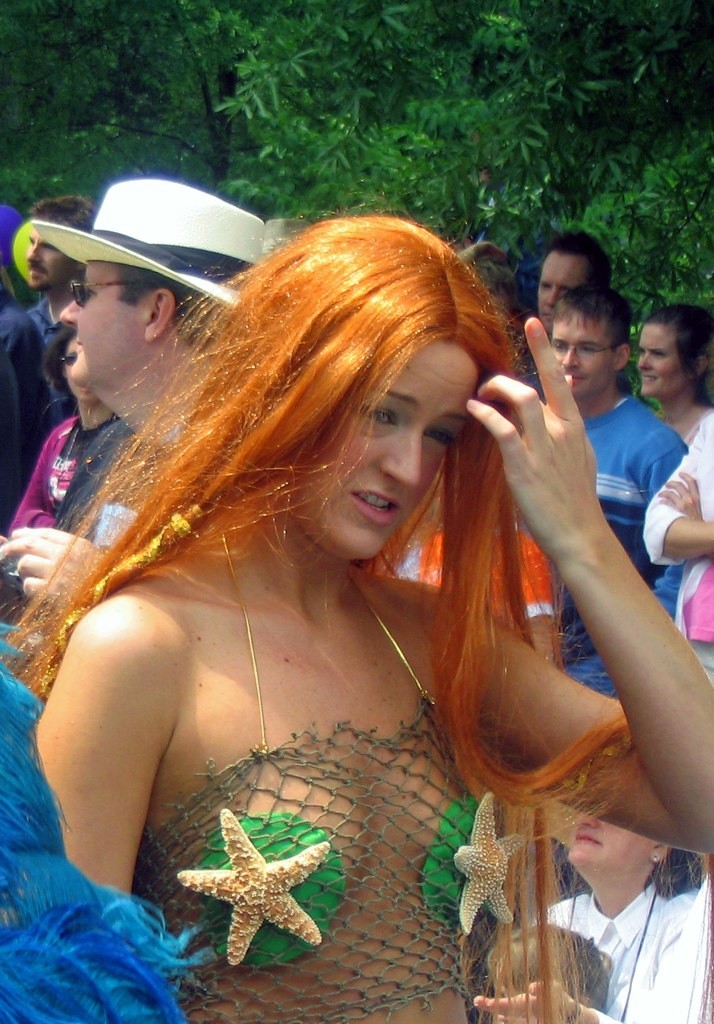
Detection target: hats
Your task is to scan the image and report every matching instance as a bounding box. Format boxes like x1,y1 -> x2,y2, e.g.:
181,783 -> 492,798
263,217 -> 313,255
31,179 -> 265,308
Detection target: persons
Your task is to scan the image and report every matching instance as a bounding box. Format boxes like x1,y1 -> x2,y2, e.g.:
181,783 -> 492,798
0,176 -> 714,1024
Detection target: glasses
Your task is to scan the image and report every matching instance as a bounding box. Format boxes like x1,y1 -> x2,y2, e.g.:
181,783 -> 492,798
58,356 -> 77,365
549,338 -> 616,360
70,279 -> 187,318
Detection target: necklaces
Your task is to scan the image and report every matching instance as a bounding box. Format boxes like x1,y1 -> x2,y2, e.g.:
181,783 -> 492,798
54,416 -> 116,500
